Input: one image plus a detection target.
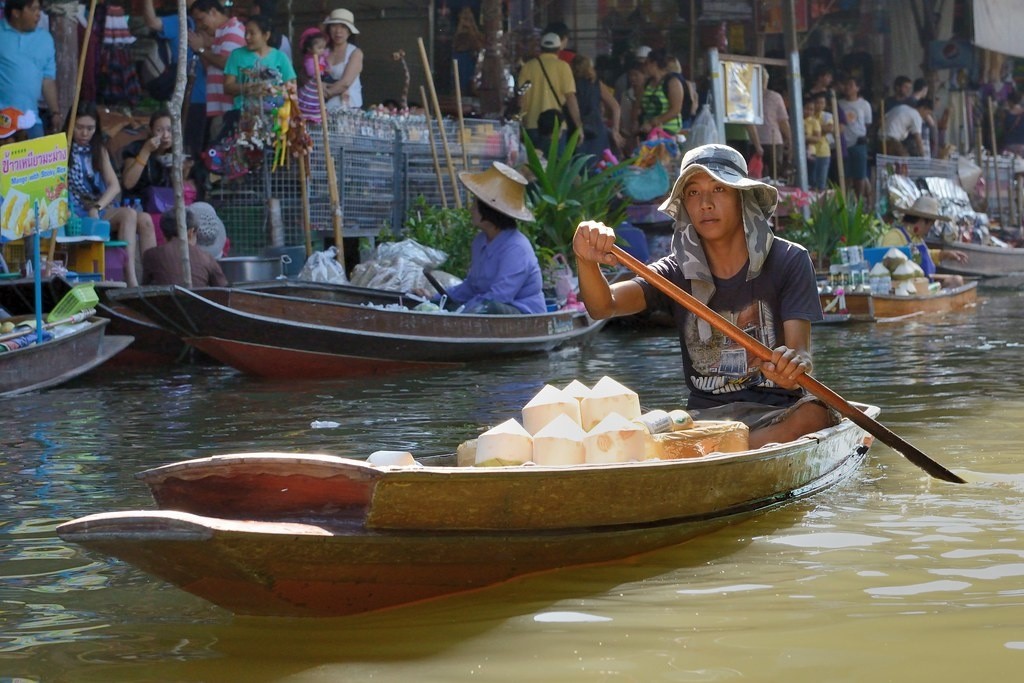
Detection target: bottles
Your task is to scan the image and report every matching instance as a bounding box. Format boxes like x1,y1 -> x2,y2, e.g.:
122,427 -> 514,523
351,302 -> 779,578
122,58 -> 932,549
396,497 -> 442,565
109,198 -> 143,214
357,103 -> 426,142
99,210 -> 106,217
470,110 -> 477,119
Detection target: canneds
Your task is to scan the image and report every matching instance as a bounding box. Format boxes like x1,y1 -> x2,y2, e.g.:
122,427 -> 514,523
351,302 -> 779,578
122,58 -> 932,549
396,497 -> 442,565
830,246 -> 869,285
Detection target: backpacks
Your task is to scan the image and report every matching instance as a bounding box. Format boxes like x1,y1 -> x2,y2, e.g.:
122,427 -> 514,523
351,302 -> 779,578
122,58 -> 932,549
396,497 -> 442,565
643,72 -> 692,123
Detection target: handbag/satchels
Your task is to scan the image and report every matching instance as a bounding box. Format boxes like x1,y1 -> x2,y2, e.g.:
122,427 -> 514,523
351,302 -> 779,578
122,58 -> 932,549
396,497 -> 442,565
560,104 -> 575,128
747,152 -> 763,178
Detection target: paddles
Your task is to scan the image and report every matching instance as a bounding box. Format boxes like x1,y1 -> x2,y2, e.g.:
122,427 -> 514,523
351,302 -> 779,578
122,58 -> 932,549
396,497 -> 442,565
423,270 -> 454,302
607,242 -> 967,483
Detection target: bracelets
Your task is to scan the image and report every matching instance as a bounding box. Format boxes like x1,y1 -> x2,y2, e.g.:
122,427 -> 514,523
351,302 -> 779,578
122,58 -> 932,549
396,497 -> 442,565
49,112 -> 61,118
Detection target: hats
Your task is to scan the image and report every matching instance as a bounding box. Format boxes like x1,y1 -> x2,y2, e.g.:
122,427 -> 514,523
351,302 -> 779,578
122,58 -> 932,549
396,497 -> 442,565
457,162 -> 538,222
323,8 -> 359,34
898,197 -> 951,222
658,144 -> 780,343
634,46 -> 652,59
184,200 -> 227,258
958,155 -> 983,196
301,28 -> 321,48
540,31 -> 561,48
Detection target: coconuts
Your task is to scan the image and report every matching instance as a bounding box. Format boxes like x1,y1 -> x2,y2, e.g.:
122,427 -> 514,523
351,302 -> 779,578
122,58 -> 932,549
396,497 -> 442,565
471,375 -> 647,470
869,248 -> 925,283
365,450 -> 414,468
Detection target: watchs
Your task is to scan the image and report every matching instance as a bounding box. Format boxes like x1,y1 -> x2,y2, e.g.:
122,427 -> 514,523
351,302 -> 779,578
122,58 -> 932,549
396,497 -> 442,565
196,47 -> 205,54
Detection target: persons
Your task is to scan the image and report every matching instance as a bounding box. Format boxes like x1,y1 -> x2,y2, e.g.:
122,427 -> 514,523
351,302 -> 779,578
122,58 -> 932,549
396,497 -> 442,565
883,197 -> 968,288
78,0 -> 292,139
517,23 -> 699,178
60,101 -> 155,287
322,9 -> 363,110
1000,93 -> 1024,157
224,15 -> 298,127
143,205 -> 228,286
877,76 -> 936,158
121,109 -> 194,246
572,144 -> 842,449
759,67 -> 792,172
408,161 -> 546,316
0,0 -> 63,145
298,28 -> 329,122
804,66 -> 871,197
726,124 -> 764,165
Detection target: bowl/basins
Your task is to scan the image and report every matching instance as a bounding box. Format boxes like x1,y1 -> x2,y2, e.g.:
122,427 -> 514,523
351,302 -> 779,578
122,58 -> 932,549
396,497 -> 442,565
217,256 -> 283,284
158,154 -> 185,167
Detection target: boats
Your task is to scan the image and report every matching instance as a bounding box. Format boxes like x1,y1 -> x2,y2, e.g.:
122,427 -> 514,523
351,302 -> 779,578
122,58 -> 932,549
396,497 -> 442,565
58,401 -> 883,619
813,276 -> 979,324
0,312 -> 133,396
48,271 -> 634,378
921,238 -> 1024,281
